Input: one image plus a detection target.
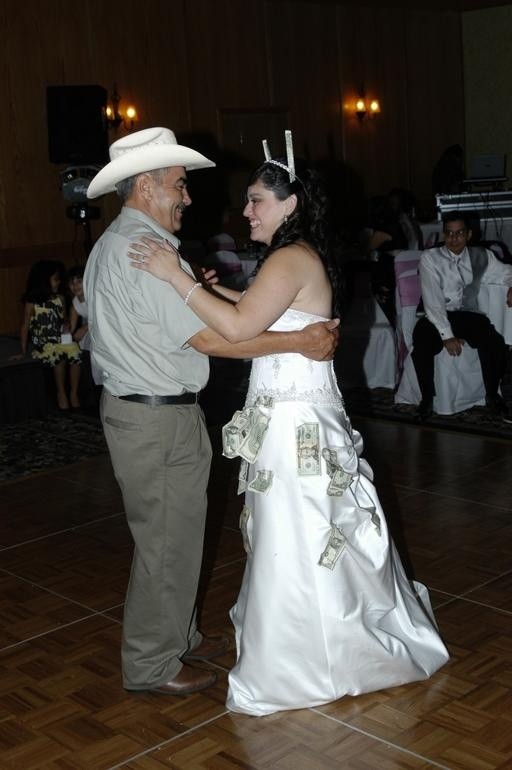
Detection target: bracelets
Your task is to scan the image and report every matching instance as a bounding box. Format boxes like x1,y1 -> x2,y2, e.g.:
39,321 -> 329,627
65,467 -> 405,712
181,282 -> 203,308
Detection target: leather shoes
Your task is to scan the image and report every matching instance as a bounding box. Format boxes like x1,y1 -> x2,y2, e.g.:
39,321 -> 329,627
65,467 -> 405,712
124,664 -> 216,696
488,395 -> 512,426
413,398 -> 433,416
182,636 -> 230,658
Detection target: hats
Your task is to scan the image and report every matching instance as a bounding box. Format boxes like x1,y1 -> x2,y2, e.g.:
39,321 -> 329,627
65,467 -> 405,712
86,126 -> 216,199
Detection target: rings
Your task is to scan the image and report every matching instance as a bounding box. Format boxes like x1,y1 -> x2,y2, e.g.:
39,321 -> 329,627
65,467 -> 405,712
139,255 -> 146,262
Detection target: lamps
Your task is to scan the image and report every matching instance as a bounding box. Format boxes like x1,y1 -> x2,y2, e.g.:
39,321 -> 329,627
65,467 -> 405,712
356,97 -> 383,123
109,81 -> 138,132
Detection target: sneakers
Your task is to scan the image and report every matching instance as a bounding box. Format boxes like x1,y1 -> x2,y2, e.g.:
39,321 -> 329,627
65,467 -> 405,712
58,397 -> 69,414
70,397 -> 80,414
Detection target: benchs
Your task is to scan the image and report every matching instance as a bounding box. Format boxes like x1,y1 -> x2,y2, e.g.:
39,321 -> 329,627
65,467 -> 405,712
0,335 -> 91,419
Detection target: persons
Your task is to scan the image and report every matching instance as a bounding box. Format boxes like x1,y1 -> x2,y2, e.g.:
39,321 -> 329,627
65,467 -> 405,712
10,259 -> 85,410
81,127 -> 342,699
66,261 -> 88,342
127,156 -> 388,716
410,211 -> 510,422
357,183 -> 422,335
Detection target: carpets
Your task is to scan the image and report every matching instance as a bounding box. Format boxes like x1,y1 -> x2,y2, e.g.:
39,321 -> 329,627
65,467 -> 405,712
0,375 -> 512,490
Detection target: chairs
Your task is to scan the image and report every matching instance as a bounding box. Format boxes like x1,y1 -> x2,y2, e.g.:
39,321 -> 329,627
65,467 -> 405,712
201,250 -> 256,393
363,298 -> 397,394
201,230 -> 239,251
394,248 -> 486,417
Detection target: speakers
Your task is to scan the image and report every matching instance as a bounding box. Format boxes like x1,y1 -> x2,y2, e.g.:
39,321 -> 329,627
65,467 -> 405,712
46,84 -> 110,167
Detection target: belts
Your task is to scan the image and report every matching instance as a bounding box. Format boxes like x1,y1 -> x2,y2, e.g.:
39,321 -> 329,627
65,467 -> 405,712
111,393 -> 199,404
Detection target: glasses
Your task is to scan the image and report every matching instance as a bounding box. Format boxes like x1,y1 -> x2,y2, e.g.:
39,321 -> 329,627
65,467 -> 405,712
444,230 -> 467,235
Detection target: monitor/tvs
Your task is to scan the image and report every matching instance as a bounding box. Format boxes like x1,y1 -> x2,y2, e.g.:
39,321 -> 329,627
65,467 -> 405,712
471,152 -> 505,180
